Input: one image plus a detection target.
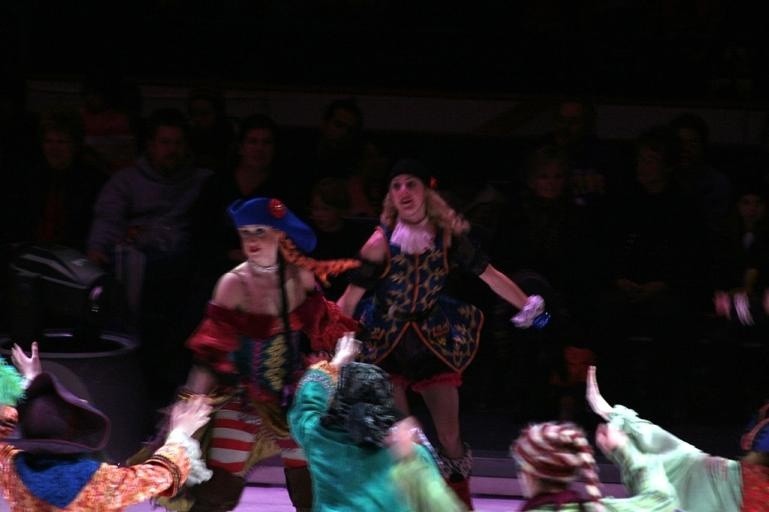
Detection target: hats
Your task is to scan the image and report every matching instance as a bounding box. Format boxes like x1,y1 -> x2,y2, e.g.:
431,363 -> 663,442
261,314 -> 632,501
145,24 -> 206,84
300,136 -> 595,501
385,156 -> 433,186
227,196 -> 318,257
0,369 -> 111,455
